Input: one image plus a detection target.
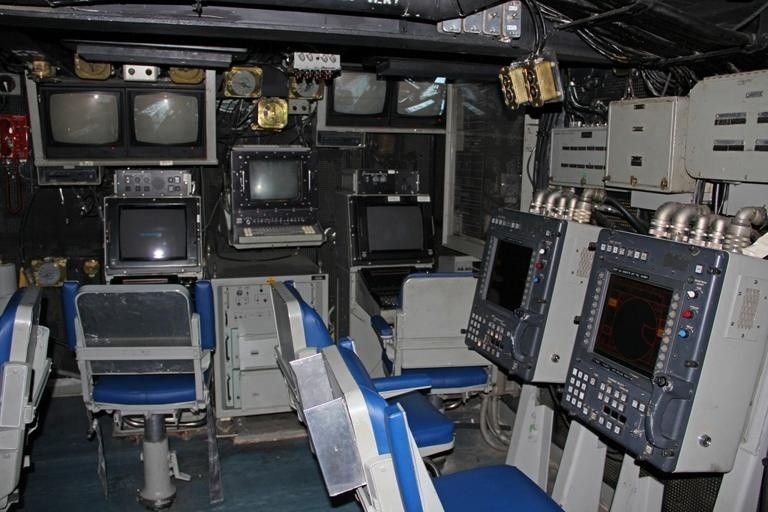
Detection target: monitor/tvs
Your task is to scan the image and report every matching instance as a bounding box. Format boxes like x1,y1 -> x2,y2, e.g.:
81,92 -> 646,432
390,78 -> 446,129
483,237 -> 534,312
38,86 -> 126,160
104,196 -> 202,273
229,151 -> 319,225
350,196 -> 434,264
126,87 -> 207,161
326,68 -> 390,128
587,270 -> 675,379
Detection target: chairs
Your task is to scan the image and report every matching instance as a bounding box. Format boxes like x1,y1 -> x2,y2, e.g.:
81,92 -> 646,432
275,339 -> 564,512
0,282 -> 56,510
265,276 -> 458,477
370,267 -> 506,449
56,272 -> 218,512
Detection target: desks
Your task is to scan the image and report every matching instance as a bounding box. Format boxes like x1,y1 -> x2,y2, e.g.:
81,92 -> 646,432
64,248 -> 331,424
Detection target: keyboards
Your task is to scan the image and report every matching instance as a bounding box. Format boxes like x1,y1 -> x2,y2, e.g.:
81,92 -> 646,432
238,227 -> 324,243
